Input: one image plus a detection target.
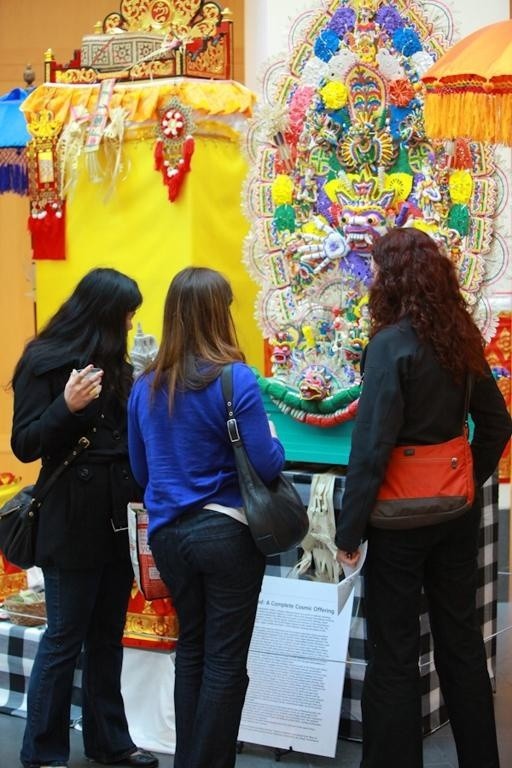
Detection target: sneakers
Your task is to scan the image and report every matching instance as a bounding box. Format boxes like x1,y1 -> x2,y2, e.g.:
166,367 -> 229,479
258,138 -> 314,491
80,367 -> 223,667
114,747 -> 159,768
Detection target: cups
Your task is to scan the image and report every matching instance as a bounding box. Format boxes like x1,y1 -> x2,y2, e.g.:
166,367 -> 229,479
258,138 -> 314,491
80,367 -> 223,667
77,367 -> 101,399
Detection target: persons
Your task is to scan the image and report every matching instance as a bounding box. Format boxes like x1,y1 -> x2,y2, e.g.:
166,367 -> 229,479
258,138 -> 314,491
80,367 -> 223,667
332,224 -> 511,767
11,268 -> 162,768
126,265 -> 285,767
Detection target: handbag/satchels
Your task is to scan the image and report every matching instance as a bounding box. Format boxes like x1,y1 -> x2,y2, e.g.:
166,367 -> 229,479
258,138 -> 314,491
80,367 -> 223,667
367,367 -> 475,530
221,363 -> 310,559
1,481 -> 39,568
